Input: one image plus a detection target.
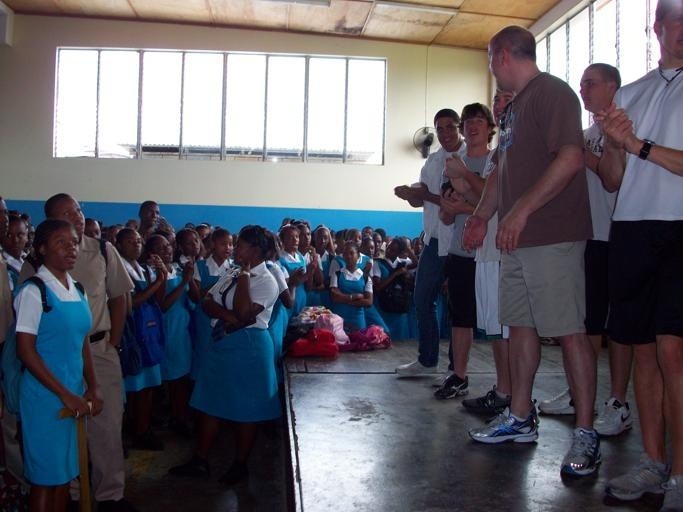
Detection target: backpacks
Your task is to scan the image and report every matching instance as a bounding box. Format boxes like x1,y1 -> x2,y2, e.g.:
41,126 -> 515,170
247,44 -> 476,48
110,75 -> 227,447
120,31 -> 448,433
377,258 -> 412,313
132,297 -> 163,366
0,276 -> 51,415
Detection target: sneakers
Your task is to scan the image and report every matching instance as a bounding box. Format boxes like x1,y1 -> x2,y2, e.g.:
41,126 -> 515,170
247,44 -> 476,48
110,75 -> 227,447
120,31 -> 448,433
433,373 -> 470,401
433,370 -> 456,386
608,453 -> 668,501
398,362 -> 439,375
591,398 -> 634,435
560,428 -> 603,476
538,387 -> 577,415
466,406 -> 539,444
660,474 -> 682,511
486,399 -> 541,427
462,385 -> 508,416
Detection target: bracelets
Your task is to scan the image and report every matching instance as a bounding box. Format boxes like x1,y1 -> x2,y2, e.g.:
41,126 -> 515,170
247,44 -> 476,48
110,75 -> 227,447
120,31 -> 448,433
240,271 -> 250,277
235,270 -> 251,276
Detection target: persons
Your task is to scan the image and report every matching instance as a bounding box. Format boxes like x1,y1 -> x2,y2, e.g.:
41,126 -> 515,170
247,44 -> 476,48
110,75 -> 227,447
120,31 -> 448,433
459,26 -> 601,477
393,109 -> 465,387
444,79 -> 520,421
171,224 -> 283,486
13,218 -> 104,511
432,103 -> 498,401
278,217 -> 449,354
596,1 -> 683,510
0,201 -> 237,464
19,194 -> 136,512
537,64 -> 634,437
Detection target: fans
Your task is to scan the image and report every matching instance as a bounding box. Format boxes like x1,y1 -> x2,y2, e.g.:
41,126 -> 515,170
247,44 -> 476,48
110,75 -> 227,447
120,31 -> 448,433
414,127 -> 441,159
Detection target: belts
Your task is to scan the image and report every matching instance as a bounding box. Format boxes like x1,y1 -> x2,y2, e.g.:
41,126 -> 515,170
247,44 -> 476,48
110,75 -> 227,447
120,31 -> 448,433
90,331 -> 105,343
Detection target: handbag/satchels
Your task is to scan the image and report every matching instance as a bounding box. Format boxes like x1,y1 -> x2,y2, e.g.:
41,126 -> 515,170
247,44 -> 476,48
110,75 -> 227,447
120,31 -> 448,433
119,322 -> 141,376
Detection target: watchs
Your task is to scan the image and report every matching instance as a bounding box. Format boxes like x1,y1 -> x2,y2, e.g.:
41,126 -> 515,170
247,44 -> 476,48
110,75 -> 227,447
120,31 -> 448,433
639,137 -> 655,161
